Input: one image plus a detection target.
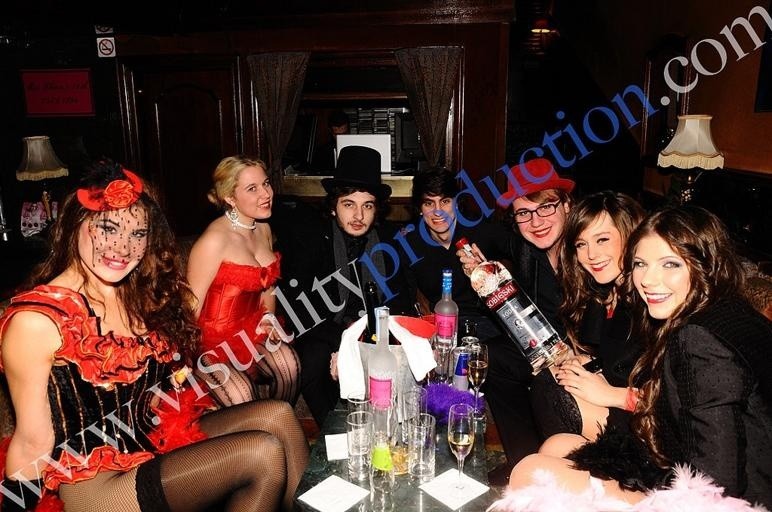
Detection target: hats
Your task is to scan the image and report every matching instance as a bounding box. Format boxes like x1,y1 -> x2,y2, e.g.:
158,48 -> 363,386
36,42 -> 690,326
320,145 -> 391,201
495,159 -> 576,209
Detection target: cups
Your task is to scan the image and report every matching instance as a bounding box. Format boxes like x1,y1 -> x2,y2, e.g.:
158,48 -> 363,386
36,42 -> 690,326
346,387 -> 436,493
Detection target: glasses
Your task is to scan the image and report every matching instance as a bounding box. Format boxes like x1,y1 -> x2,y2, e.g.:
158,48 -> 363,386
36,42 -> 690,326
511,200 -> 562,224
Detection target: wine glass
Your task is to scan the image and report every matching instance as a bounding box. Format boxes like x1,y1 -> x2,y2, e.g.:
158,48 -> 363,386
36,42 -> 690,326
467,343 -> 488,416
447,404 -> 477,492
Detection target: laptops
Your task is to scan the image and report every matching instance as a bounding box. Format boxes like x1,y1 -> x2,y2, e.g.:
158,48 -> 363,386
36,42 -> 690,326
335,133 -> 392,174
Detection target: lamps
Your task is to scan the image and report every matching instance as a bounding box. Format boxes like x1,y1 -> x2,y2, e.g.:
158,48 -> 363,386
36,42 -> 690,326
655,108 -> 730,208
16,134 -> 71,227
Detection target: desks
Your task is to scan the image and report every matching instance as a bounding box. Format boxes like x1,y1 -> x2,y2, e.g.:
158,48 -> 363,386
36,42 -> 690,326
294,363 -> 509,511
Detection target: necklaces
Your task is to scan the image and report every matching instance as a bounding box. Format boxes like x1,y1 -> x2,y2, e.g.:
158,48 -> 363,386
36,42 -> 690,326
224,210 -> 257,230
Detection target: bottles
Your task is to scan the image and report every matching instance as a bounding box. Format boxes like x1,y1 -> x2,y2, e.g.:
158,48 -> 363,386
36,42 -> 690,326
368,305 -> 396,436
364,280 -> 383,342
455,237 -> 568,368
434,269 -> 458,378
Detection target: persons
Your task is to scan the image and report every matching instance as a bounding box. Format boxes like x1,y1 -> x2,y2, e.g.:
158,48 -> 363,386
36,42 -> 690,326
185,153 -> 301,409
0,161 -> 311,511
508,205 -> 771,512
